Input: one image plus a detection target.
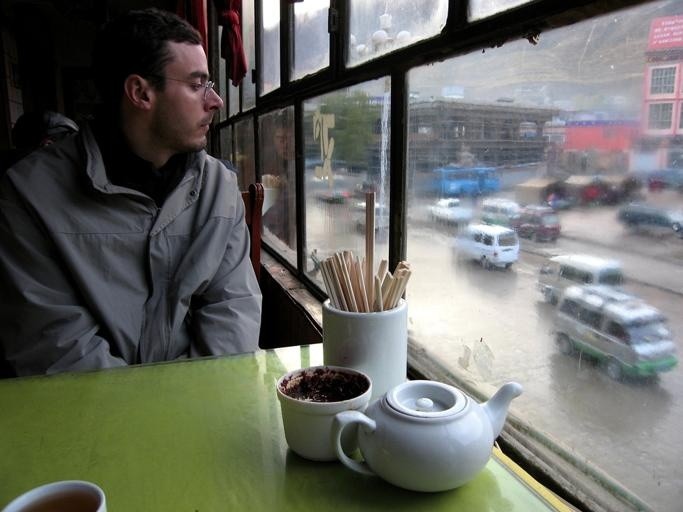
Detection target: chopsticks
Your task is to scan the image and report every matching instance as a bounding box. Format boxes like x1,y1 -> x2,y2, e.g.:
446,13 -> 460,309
313,191 -> 413,313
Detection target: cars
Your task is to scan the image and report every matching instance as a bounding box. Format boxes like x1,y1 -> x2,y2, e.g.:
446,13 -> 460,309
615,197 -> 682,235
429,197 -> 563,241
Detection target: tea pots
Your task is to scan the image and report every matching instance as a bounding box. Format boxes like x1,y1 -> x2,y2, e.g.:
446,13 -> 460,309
330,378 -> 523,493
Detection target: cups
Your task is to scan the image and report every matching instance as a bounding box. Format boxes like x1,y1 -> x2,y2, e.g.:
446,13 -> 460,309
320,299 -> 410,376
0,478 -> 106,512
275,364 -> 373,462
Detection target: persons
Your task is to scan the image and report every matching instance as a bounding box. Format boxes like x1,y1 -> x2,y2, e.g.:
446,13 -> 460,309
11,108 -> 80,159
0,8 -> 262,375
264,110 -> 296,251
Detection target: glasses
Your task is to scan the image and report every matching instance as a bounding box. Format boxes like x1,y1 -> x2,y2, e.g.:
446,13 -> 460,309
148,74 -> 215,100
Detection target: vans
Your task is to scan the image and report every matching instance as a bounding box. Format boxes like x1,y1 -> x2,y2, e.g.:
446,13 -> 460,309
348,202 -> 390,232
455,220 -> 517,270
537,252 -> 679,383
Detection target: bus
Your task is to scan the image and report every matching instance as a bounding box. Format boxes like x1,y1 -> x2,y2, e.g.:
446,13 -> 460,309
431,164 -> 498,195
